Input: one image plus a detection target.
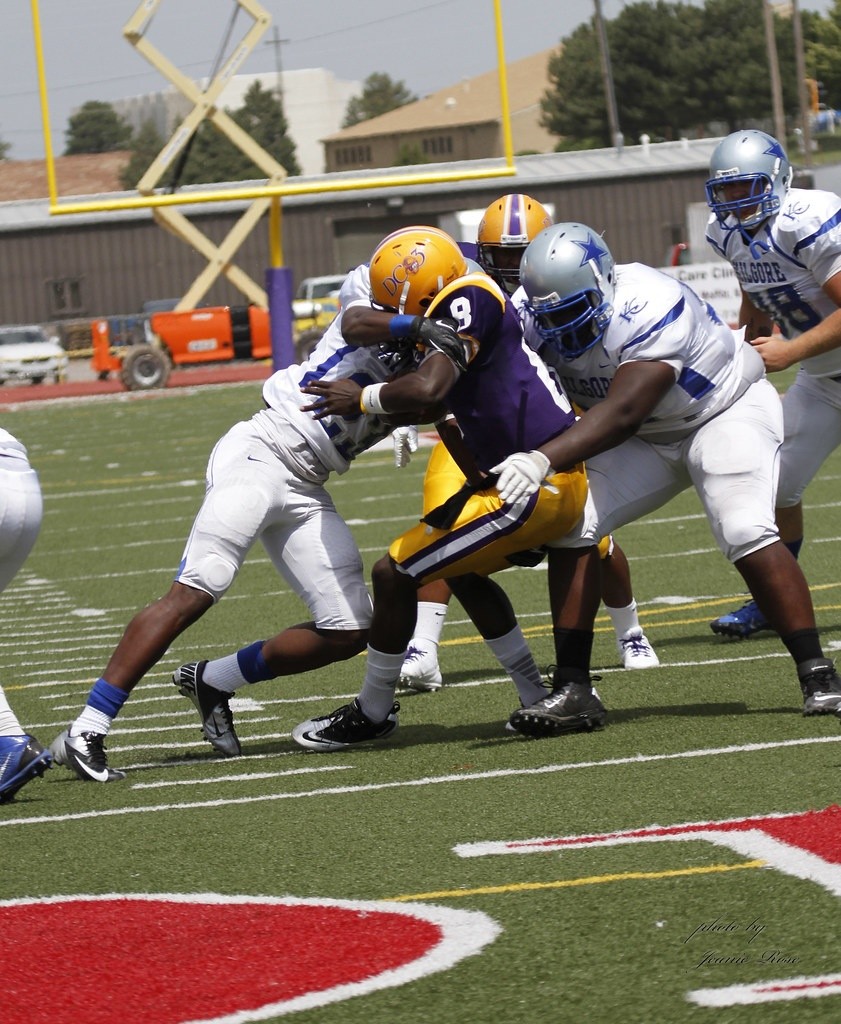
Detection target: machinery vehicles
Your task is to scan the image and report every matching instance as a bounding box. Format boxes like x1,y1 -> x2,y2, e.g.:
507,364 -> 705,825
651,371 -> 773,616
91,0 -> 288,390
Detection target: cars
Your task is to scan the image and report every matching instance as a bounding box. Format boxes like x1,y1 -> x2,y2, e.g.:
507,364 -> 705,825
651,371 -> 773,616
0,326 -> 68,386
290,274 -> 350,319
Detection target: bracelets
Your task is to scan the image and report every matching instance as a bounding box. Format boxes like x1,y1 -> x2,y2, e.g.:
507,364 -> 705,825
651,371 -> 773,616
362,382 -> 392,414
359,393 -> 368,414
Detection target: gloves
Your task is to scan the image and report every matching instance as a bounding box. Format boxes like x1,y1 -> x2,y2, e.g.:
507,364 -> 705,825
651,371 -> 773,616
391,421 -> 418,468
488,448 -> 549,507
392,310 -> 468,373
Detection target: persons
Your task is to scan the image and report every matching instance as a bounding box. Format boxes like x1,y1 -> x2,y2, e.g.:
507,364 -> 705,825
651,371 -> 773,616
293,227 -> 590,752
0,424 -> 52,803
50,261 -> 471,782
707,130 -> 841,640
392,192 -> 841,734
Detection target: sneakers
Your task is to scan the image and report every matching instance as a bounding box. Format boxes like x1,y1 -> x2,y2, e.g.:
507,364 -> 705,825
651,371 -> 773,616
617,634 -> 659,671
172,657 -> 242,758
709,592 -> 778,640
0,733 -> 56,799
292,694 -> 413,752
509,664 -> 607,738
48,726 -> 129,784
396,638 -> 443,695
795,656 -> 841,716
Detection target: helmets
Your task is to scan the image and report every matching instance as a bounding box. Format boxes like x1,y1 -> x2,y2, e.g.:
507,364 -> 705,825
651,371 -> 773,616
477,193 -> 551,298
519,221 -> 618,357
369,226 -> 467,360
705,129 -> 791,231
